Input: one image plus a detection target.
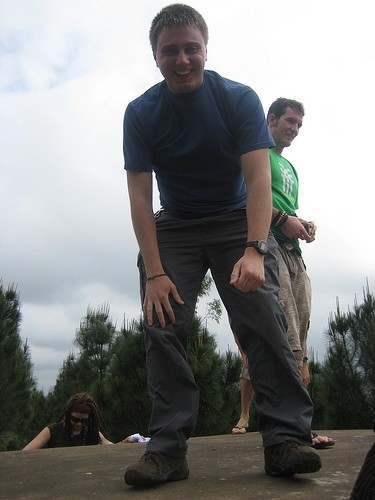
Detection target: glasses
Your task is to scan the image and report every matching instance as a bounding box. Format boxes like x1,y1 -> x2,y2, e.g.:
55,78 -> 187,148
71,414 -> 89,423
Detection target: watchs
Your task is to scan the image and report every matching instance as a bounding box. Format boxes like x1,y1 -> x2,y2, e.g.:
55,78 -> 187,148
244,240 -> 268,254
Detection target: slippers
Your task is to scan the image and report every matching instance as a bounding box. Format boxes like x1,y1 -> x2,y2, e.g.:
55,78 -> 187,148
231,425 -> 250,434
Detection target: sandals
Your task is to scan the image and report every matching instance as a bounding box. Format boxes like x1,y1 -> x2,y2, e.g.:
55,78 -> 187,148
310,432 -> 335,449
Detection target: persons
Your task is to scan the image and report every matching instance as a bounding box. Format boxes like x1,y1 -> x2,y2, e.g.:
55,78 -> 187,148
21,393 -> 146,451
231,98 -> 337,450
124,3 -> 322,484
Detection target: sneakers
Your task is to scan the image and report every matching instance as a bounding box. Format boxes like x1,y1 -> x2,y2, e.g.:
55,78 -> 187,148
124,452 -> 188,486
264,439 -> 321,476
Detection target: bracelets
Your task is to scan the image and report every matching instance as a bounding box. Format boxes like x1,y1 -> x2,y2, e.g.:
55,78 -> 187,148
270,211 -> 288,230
146,273 -> 167,280
